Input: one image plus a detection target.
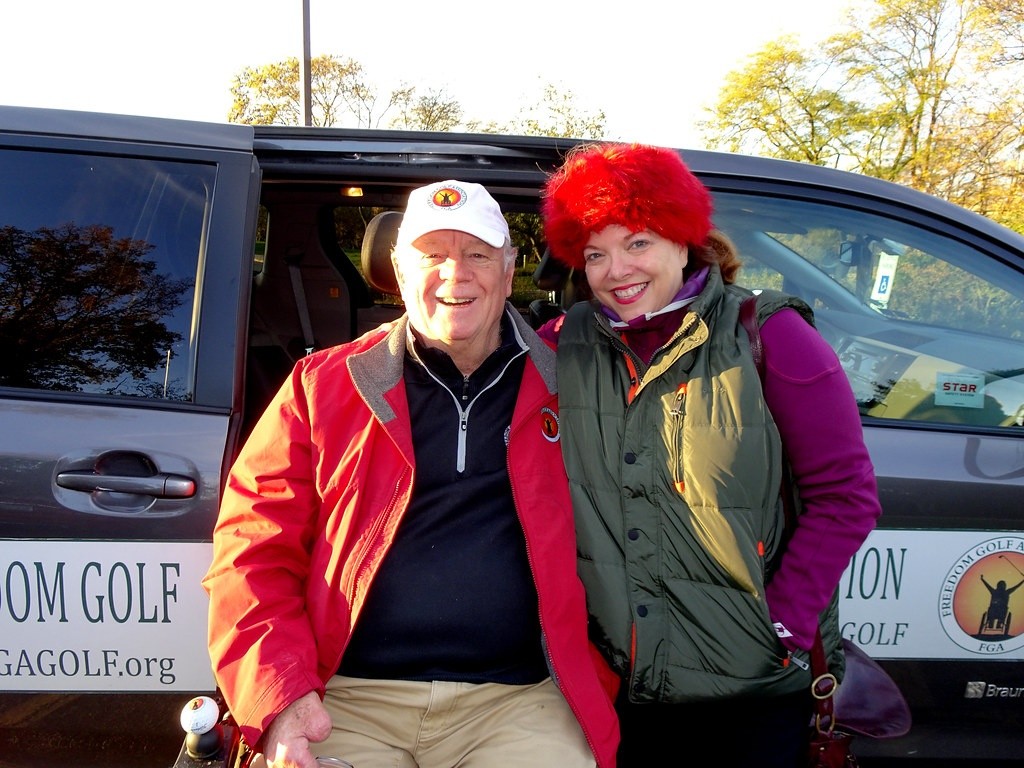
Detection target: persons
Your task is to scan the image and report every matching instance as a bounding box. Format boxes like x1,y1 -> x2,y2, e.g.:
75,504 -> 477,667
544,142 -> 883,768
201,180 -> 620,768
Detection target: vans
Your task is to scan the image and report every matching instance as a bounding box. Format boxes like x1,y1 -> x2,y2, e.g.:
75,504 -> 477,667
0,104 -> 1024,768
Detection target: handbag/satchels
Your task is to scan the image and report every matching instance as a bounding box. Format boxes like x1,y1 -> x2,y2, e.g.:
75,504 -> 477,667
810,638 -> 916,767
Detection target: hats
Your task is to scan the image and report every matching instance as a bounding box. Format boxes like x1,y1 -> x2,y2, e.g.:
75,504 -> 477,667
397,179 -> 511,248
